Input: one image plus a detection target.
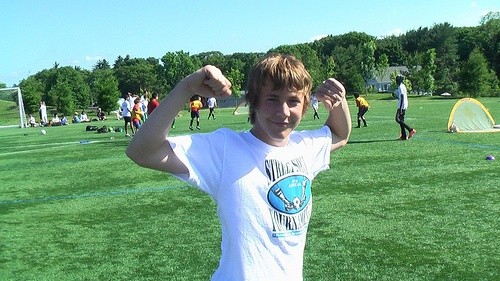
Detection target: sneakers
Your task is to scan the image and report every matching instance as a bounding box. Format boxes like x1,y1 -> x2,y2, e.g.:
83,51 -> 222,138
397,135 -> 408,140
408,129 -> 416,139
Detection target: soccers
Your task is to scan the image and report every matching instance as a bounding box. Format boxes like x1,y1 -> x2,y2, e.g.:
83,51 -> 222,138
41,130 -> 46,134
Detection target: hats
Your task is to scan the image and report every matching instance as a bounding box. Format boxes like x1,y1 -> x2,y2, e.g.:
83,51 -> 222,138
74,112 -> 79,114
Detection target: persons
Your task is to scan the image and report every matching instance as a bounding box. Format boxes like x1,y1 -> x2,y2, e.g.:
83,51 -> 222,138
27,101 -> 68,127
80,111 -> 92,122
310,91 -> 320,120
73,112 -> 80,123
396,76 -> 416,140
207,97 -> 217,120
97,110 -> 106,121
116,92 -> 175,137
189,97 -> 202,131
354,93 -> 370,128
125,53 -> 352,281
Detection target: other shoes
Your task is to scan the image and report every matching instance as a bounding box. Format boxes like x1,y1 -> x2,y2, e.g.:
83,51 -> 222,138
125,134 -> 130,137
172,126 -> 176,129
131,135 -> 134,139
189,126 -> 193,131
357,126 -> 360,128
196,126 -> 200,130
363,125 -> 367,127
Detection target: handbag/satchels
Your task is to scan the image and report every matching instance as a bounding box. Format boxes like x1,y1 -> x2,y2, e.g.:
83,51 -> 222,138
86,126 -> 97,131
97,125 -> 123,133
